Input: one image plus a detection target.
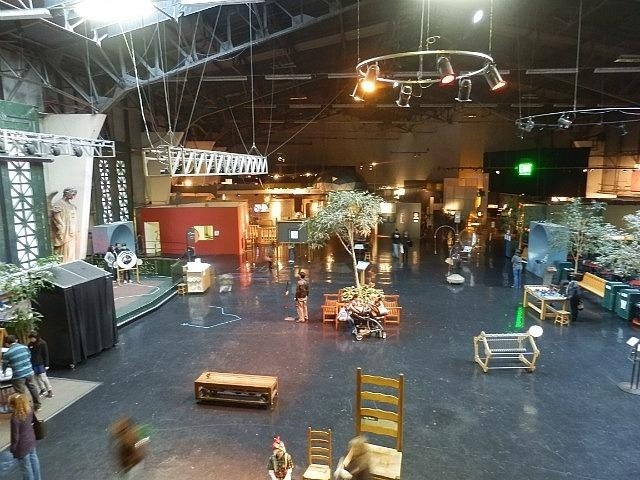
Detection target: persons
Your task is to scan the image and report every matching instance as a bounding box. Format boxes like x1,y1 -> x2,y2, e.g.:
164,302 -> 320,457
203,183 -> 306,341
391,228 -> 400,258
6,392 -> 43,480
401,230 -> 412,261
0,334 -> 42,414
116,243 -> 125,254
368,292 -> 383,329
292,271 -> 311,324
26,329 -> 55,399
266,436 -> 295,480
50,184 -> 83,263
336,294 -> 364,335
102,243 -> 119,283
122,243 -> 133,284
332,435 -> 376,480
557,279 -> 585,323
510,248 -> 524,289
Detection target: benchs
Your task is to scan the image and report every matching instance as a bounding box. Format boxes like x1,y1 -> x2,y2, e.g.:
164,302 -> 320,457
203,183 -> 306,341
573,271 -> 609,302
335,301 -> 387,332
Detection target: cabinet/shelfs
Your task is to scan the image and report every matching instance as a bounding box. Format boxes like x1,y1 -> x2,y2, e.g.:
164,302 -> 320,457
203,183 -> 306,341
186,263 -> 212,293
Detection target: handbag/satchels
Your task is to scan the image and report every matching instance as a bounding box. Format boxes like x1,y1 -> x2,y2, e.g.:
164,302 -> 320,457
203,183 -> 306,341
33,411 -> 45,440
577,303 -> 584,311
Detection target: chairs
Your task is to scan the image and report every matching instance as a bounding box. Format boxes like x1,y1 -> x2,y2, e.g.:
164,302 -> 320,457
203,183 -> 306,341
300,425 -> 334,479
344,366 -> 405,479
320,291 -> 339,325
381,293 -> 403,326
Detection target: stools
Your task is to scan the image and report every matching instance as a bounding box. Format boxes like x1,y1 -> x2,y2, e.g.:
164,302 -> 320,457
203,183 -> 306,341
177,283 -> 187,296
553,310 -> 572,328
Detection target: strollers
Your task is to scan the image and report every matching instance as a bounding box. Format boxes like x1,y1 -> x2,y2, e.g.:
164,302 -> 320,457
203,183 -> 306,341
339,300 -> 389,342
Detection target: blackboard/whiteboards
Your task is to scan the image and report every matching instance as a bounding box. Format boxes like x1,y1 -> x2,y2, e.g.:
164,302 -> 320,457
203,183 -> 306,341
276,222 -> 309,244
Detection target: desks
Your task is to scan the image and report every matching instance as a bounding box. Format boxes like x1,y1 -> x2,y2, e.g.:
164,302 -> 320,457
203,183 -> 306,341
194,370 -> 280,409
523,285 -> 569,322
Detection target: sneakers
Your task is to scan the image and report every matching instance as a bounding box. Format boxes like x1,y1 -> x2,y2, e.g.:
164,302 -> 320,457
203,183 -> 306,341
40,389 -> 54,398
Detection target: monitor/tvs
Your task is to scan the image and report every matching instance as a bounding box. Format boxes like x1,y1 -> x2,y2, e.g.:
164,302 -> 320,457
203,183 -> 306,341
253,203 -> 270,213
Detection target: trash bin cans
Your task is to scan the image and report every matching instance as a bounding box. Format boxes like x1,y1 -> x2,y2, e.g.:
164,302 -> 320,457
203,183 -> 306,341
602,281 -> 640,322
554,261 -> 572,285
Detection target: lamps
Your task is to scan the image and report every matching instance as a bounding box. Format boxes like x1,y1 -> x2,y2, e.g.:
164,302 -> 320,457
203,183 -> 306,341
350,0 -> 506,110
0,138 -> 83,158
508,0 -> 640,138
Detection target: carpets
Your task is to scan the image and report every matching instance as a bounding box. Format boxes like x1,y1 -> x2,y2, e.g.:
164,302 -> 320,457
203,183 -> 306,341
0,372 -> 103,459
